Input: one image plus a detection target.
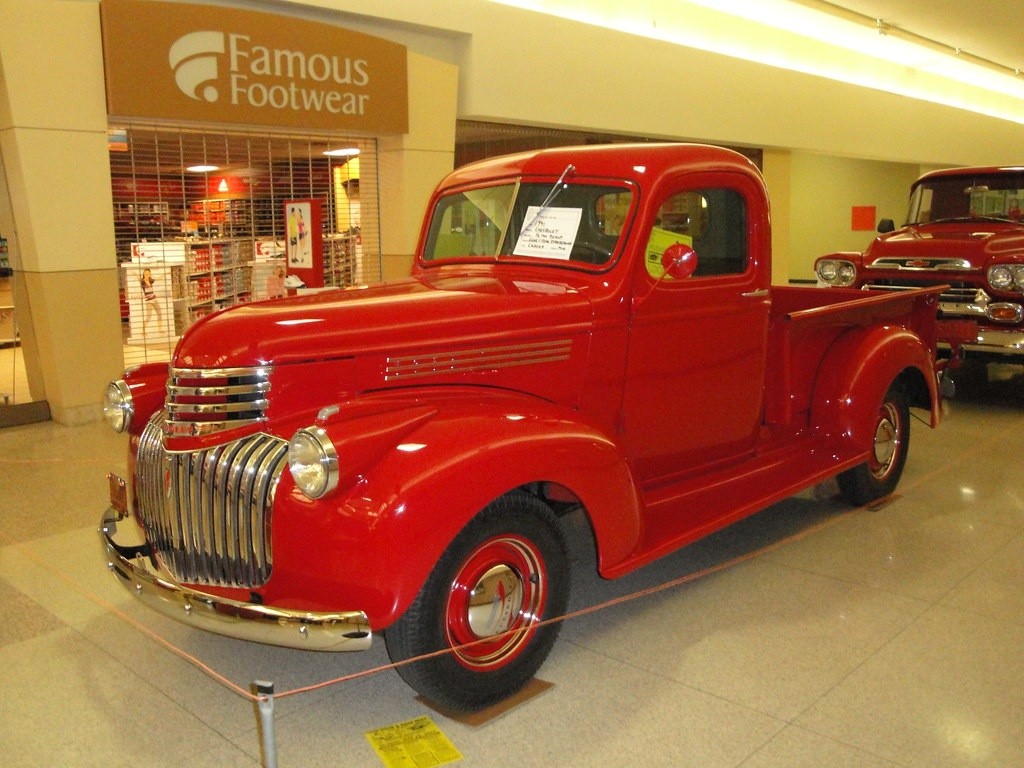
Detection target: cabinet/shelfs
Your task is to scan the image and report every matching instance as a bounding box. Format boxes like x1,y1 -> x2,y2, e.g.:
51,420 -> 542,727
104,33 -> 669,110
121,263 -> 186,345
247,234 -> 358,300
130,236 -> 278,325
114,192 -> 330,267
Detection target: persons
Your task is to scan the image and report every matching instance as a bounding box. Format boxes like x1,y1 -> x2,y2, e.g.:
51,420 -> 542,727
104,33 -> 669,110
132,269 -> 167,335
267,266 -> 285,300
288,207 -> 309,264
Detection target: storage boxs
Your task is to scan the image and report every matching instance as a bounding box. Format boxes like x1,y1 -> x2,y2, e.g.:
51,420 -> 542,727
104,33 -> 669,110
111,195 -> 356,335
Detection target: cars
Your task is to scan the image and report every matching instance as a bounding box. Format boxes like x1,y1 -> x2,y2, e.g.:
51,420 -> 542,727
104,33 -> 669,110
812,166 -> 1024,399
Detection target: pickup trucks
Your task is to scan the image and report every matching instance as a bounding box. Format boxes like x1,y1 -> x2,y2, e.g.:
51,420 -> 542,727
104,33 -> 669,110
99,142 -> 953,713
